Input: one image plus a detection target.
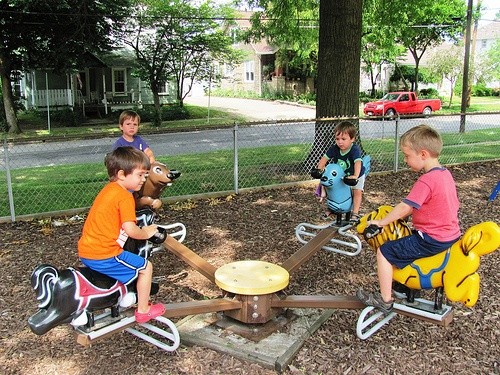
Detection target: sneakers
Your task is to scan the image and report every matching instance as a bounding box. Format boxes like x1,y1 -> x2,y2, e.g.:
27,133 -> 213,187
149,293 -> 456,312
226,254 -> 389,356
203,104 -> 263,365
357,289 -> 396,313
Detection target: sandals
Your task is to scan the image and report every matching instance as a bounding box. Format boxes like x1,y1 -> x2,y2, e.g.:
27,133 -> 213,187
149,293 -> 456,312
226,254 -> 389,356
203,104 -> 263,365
135,303 -> 165,323
349,214 -> 361,226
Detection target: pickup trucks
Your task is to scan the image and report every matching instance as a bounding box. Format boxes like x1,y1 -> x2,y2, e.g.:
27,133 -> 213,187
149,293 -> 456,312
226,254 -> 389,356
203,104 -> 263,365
363,91 -> 442,121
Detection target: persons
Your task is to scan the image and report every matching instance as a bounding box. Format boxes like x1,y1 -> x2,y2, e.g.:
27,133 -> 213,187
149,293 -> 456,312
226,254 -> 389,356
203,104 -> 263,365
356,125 -> 462,314
317,121 -> 365,225
112,110 -> 155,193
78,147 -> 166,322
